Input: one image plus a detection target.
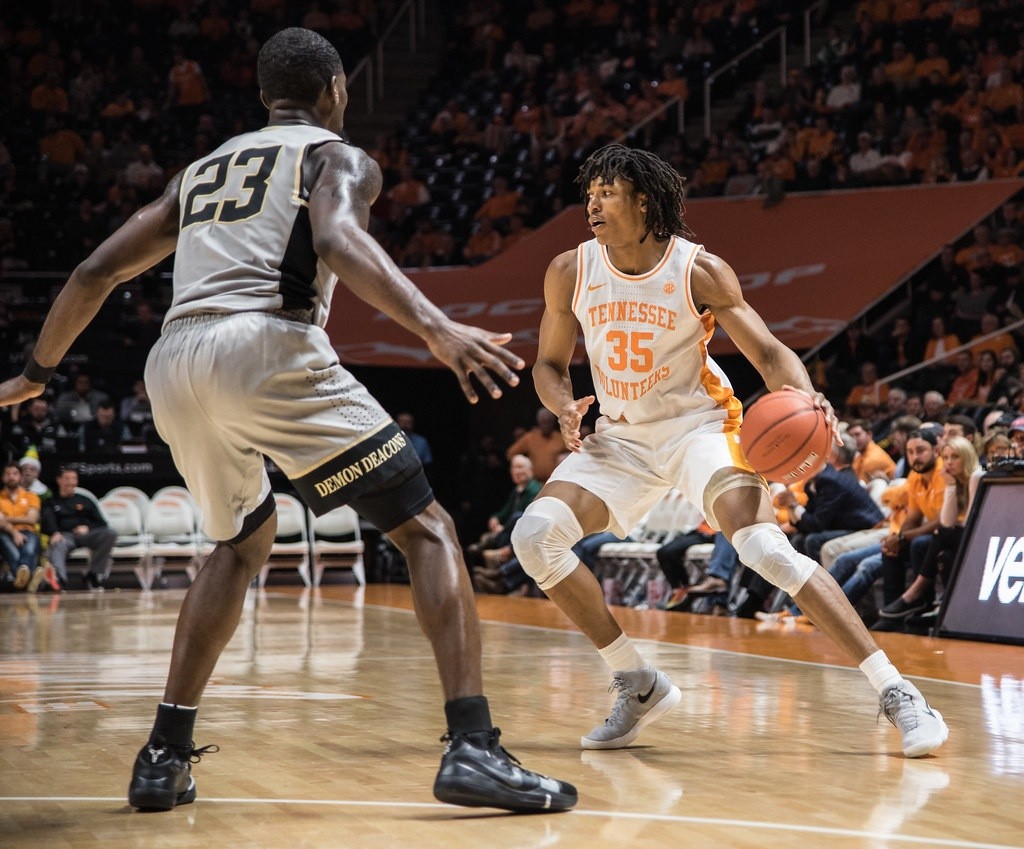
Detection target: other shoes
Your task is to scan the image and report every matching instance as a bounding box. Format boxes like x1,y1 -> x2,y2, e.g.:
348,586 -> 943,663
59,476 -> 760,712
14,565 -> 31,588
26,567 -> 44,592
465,558 -> 812,632
44,564 -> 63,591
84,571 -> 104,592
919,591 -> 945,617
878,596 -> 929,617
870,617 -> 906,633
0,563 -> 15,581
903,610 -> 937,629
56,572 -> 70,590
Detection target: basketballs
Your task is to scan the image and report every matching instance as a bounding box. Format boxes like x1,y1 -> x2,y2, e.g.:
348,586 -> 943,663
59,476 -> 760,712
741,391 -> 833,485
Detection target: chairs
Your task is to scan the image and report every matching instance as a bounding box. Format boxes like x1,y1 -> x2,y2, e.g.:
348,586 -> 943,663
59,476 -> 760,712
48,487 -> 367,586
596,543 -> 716,615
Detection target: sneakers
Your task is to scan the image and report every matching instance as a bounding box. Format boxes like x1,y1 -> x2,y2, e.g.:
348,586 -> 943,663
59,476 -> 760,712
433,727 -> 578,812
128,739 -> 219,810
874,679 -> 948,758
581,667 -> 681,748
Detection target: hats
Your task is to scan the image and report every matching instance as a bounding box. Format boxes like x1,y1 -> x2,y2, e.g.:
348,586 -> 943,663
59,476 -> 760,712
1007,416 -> 1024,439
17,444 -> 42,470
989,412 -> 1015,430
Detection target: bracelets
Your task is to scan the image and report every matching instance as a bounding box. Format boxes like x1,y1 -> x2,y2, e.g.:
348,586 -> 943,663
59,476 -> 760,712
23,354 -> 66,385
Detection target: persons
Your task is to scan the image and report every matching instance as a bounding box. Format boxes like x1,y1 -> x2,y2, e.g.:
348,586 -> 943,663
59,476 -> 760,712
0,26 -> 578,813
512,145 -> 948,757
0,0 -> 1024,647
